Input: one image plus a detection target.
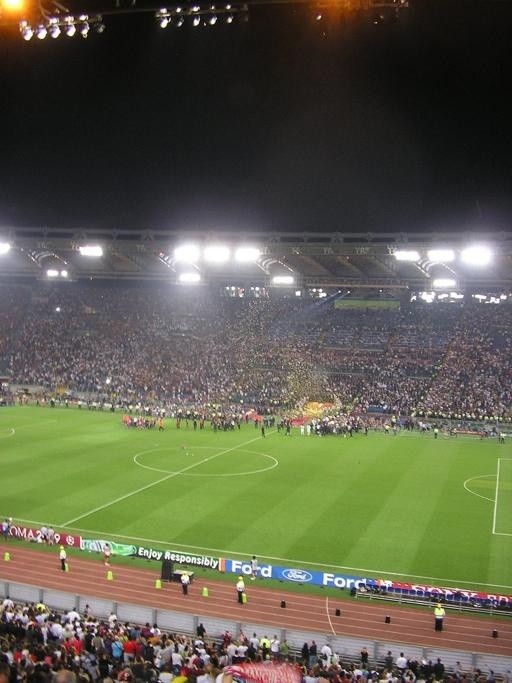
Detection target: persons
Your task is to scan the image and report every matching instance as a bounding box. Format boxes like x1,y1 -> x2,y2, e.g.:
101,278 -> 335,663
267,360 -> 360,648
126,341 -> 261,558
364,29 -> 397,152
40,524 -> 48,543
1,276 -> 512,444
181,571 -> 190,594
0,592 -> 512,683
59,544 -> 66,571
103,542 -> 110,565
1,518 -> 10,540
235,574 -> 245,603
48,525 -> 54,546
250,555 -> 258,580
